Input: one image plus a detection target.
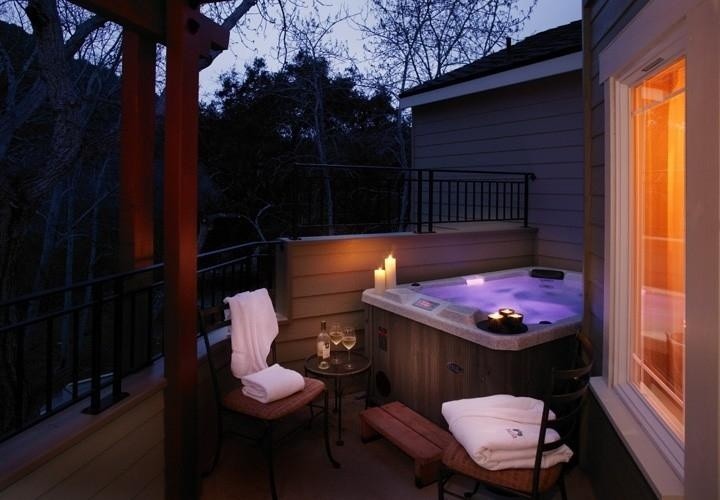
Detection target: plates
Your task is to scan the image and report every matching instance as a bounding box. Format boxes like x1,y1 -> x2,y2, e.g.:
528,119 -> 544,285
476,320 -> 528,335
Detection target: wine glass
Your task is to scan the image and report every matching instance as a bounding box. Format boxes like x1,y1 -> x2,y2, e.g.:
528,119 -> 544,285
342,326 -> 357,371
328,321 -> 345,365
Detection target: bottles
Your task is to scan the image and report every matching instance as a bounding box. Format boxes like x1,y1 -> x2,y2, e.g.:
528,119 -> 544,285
316,319 -> 331,370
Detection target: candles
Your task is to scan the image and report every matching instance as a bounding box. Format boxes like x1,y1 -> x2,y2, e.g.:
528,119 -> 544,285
385,253 -> 397,290
374,265 -> 385,292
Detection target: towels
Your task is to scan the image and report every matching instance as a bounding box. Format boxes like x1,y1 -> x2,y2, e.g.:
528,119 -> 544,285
240,363 -> 305,404
223,288 -> 280,380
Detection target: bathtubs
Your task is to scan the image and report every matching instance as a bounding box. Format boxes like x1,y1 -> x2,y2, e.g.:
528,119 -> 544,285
413,271 -> 584,324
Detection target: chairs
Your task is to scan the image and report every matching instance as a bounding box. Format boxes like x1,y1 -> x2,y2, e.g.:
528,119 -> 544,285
196,304 -> 340,499
438,332 -> 597,500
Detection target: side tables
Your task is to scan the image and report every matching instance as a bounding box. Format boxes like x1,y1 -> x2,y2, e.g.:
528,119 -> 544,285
305,350 -> 373,447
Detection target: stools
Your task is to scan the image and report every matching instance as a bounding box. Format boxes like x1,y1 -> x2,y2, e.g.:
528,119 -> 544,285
359,400 -> 453,489
